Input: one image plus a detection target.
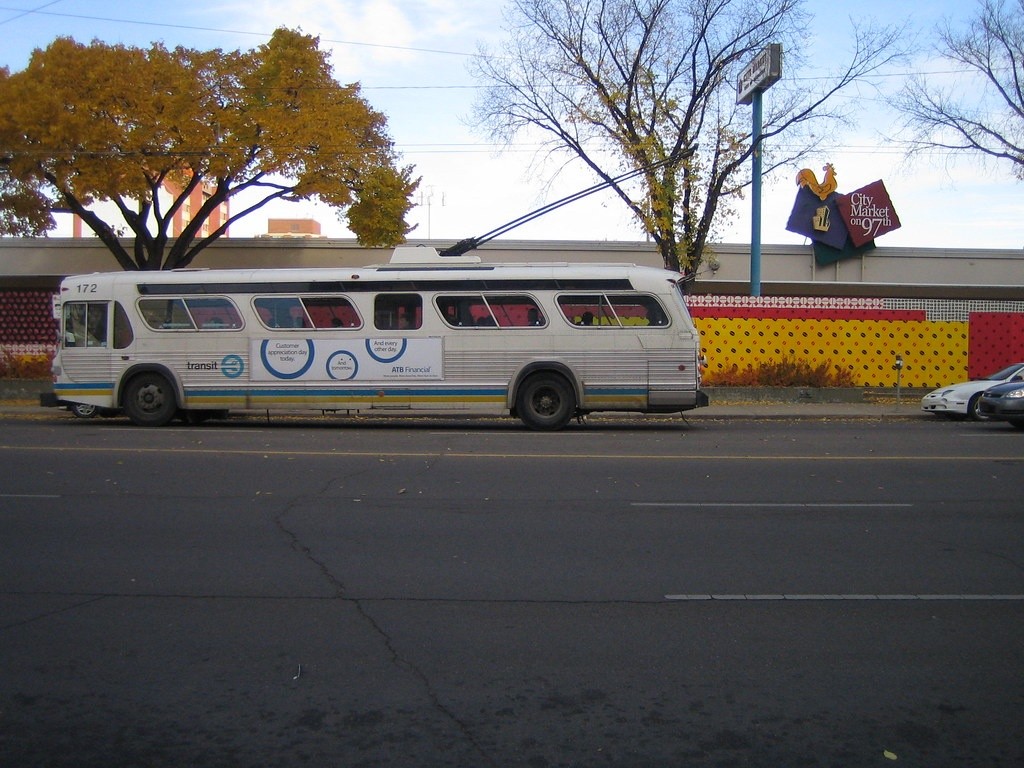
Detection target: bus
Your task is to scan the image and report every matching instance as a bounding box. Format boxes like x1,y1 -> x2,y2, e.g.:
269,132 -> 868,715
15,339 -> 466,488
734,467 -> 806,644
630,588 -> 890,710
50,144 -> 709,433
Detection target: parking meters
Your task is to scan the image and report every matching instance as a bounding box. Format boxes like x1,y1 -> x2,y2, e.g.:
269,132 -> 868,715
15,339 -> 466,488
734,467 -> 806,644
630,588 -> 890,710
894,354 -> 904,404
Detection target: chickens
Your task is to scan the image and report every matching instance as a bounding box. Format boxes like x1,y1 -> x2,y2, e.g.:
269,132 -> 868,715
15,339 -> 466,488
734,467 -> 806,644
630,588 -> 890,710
795,163 -> 838,201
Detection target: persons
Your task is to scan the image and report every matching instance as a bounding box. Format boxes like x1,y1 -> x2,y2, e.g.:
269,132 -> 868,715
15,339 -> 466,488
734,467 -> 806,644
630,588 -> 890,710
645,311 -> 656,326
398,313 -> 413,329
575,311 -> 595,326
527,308 -> 541,326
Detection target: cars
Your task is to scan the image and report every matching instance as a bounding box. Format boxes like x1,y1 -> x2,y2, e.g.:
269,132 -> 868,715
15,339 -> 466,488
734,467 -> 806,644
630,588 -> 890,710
978,379 -> 1024,430
920,362 -> 1024,422
